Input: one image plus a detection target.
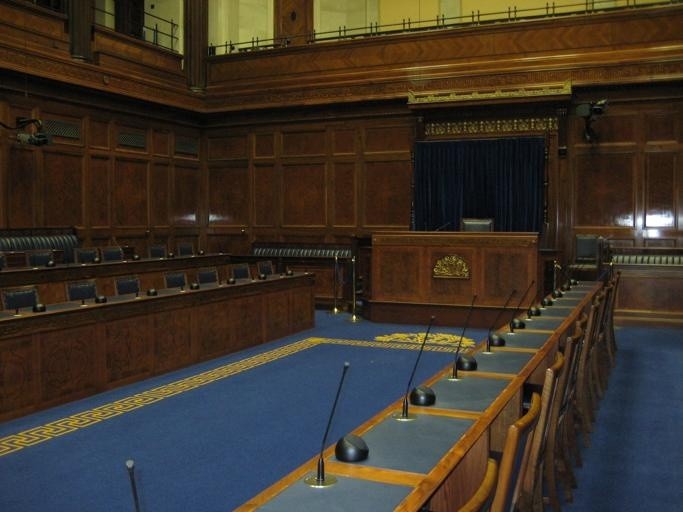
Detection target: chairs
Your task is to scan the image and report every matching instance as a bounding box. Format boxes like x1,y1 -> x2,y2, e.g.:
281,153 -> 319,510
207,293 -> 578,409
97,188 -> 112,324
461,219 -> 494,234
0,239 -> 275,309
570,234 -> 597,281
609,238 -> 634,249
643,238 -> 675,247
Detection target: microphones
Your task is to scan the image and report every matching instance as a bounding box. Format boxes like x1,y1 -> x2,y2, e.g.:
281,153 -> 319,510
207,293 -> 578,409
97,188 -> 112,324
446,293 -> 478,382
303,361 -> 350,488
394,315 -> 437,422
126,459 -> 140,512
482,289 -> 517,355
505,280 -> 535,335
524,256 -> 585,321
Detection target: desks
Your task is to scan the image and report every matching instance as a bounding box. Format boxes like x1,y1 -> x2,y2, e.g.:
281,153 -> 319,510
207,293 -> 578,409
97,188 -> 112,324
227,280 -> 604,512
0,271 -> 316,425
607,247 -> 683,329
248,244 -> 349,311
